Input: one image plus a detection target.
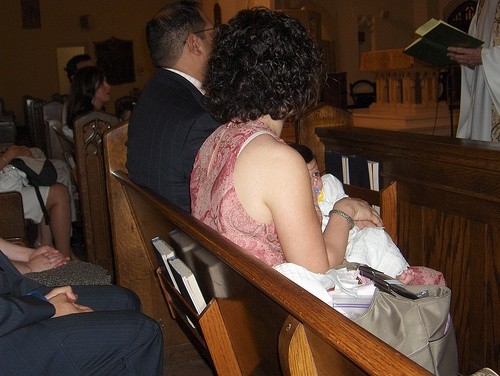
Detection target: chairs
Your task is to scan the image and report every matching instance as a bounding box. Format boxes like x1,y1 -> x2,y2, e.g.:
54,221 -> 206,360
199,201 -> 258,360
346,80 -> 377,109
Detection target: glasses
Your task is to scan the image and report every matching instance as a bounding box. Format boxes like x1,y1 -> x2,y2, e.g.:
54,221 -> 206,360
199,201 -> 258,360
183,23 -> 219,45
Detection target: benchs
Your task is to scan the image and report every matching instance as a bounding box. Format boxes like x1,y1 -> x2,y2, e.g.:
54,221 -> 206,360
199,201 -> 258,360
0,96 -> 500,376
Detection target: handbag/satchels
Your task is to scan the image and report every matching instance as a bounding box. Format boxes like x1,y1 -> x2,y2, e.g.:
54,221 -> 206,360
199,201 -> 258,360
9,157 -> 57,186
326,263 -> 458,376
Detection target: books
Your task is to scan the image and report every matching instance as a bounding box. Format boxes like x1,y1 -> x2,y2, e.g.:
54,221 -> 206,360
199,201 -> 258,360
403,19 -> 484,70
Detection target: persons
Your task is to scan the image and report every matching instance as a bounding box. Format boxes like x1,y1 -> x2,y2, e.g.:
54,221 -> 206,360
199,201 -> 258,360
127,6 -> 223,214
0,54 -> 166,376
447,0 -> 500,143
287,143 -> 411,278
193,6 -> 385,273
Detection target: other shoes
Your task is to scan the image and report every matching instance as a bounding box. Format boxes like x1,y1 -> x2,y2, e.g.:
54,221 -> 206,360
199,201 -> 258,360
33,240 -> 41,249
70,236 -> 81,248
